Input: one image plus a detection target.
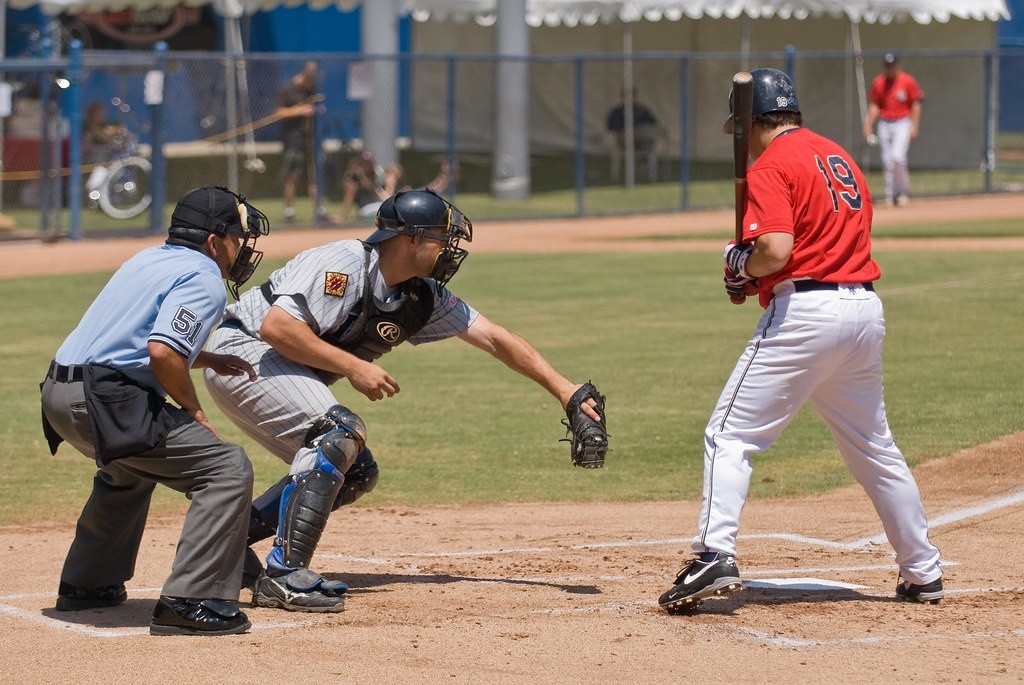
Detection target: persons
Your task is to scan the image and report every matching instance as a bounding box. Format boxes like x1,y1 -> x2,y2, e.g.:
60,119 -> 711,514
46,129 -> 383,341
274,60 -> 331,219
338,155 -> 460,223
604,83 -> 661,184
864,54 -> 923,207
657,69 -> 946,611
81,102 -> 133,151
39,186 -> 270,635
203,188 -> 606,611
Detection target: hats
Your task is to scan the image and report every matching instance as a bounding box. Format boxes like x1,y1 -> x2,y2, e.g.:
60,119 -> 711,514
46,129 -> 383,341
883,54 -> 901,65
171,186 -> 260,238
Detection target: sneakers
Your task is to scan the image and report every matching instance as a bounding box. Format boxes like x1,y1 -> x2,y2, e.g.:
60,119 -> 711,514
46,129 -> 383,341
251,568 -> 345,613
658,552 -> 743,610
895,580 -> 944,601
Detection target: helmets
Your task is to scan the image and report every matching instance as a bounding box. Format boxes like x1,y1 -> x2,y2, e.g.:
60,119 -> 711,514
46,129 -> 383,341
365,191 -> 449,243
722,68 -> 801,134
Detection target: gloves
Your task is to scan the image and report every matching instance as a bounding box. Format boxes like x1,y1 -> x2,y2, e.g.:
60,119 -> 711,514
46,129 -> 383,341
723,240 -> 760,296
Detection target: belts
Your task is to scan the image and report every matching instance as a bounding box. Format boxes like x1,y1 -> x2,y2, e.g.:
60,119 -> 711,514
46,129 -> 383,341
773,279 -> 875,291
216,318 -> 242,329
48,361 -> 83,382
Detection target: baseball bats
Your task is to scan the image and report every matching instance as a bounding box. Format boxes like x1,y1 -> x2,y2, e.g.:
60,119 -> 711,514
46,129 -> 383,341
730,70 -> 755,305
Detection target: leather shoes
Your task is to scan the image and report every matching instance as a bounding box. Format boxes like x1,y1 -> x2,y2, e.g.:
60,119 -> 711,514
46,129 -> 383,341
149,596 -> 252,636
56,581 -> 128,611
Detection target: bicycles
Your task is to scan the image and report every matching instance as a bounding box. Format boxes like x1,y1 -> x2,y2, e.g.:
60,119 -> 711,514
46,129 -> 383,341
76,100 -> 152,220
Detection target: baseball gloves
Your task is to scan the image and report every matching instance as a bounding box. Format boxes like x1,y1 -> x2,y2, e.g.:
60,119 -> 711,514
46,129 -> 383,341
557,377 -> 614,469
